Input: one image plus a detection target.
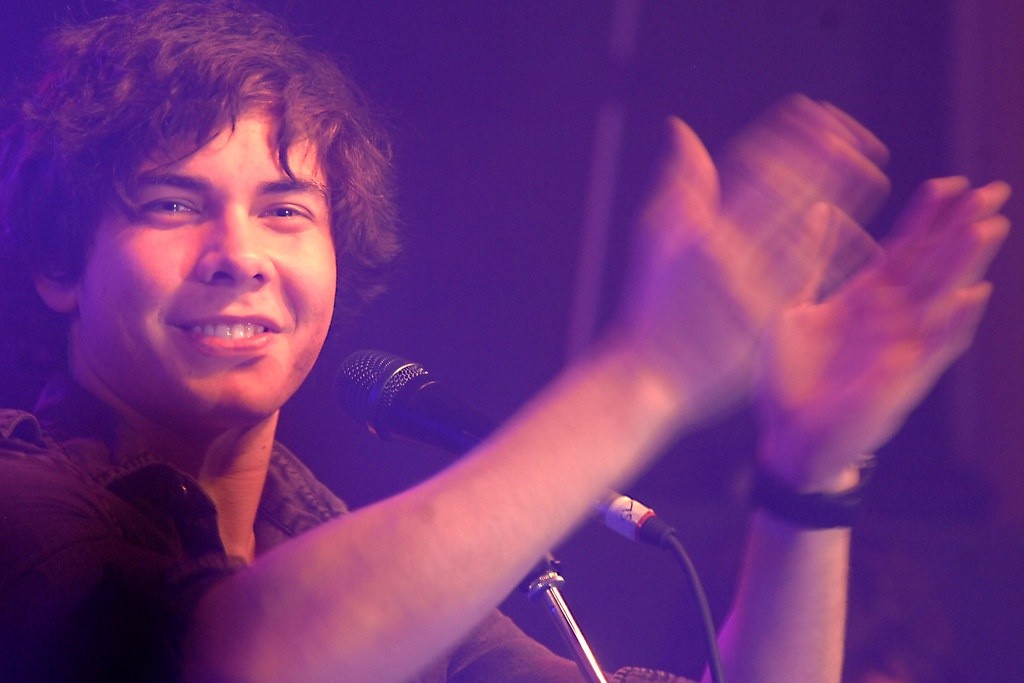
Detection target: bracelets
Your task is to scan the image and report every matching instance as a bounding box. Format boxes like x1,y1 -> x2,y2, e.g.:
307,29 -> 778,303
749,463 -> 884,527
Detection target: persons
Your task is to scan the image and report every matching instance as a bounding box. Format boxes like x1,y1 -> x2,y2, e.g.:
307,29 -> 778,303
0,0 -> 1010,683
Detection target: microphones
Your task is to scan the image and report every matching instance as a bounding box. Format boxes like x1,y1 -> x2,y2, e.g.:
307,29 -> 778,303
332,351 -> 677,549
